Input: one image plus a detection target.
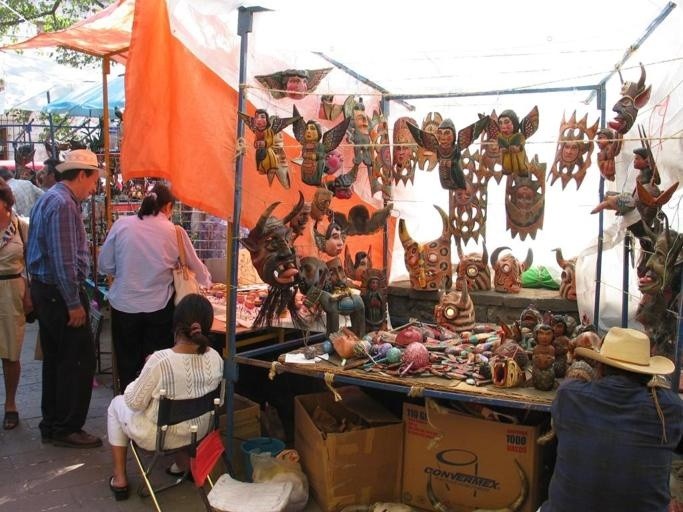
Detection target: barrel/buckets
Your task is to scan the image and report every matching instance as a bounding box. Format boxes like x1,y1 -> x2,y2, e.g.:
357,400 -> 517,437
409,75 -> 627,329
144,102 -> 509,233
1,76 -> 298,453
240,436 -> 286,482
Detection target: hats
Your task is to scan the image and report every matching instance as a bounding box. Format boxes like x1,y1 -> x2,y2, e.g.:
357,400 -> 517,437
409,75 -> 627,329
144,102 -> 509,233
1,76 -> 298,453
576,326 -> 676,375
55,149 -> 101,174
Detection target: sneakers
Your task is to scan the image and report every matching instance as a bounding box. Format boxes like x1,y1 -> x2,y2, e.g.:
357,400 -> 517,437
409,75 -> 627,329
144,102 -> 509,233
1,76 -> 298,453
3,411 -> 19,430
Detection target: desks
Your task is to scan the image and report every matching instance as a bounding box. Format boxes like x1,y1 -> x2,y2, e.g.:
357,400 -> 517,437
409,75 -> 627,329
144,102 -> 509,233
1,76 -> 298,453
277,341 -> 562,413
211,284 -> 314,337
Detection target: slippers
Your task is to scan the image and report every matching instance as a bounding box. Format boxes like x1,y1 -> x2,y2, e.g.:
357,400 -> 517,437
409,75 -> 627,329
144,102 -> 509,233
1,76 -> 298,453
109,475 -> 129,500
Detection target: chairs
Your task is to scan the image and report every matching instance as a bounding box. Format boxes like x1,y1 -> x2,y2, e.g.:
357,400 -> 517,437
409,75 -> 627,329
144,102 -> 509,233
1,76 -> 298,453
129,379 -> 235,512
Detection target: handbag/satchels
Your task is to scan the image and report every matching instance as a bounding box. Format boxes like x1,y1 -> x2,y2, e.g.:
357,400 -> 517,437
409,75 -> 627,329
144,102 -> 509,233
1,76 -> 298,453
22,279 -> 37,323
172,264 -> 200,306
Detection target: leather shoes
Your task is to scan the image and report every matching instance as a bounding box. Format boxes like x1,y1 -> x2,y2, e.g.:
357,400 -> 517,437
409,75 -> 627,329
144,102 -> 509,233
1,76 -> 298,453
41,428 -> 103,449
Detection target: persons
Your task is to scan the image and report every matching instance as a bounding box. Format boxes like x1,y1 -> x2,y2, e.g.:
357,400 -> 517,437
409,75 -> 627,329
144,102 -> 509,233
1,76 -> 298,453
1,179 -> 31,430
537,328 -> 683,510
39,160 -> 59,188
113,174 -> 123,190
1,165 -> 42,218
98,184 -> 212,394
107,294 -> 224,501
27,149 -> 102,449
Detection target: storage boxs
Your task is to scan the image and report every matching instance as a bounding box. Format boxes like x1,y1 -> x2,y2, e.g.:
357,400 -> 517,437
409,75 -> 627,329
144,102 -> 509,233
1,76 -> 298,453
294,386 -> 404,511
401,400 -> 546,512
216,392 -> 261,475
